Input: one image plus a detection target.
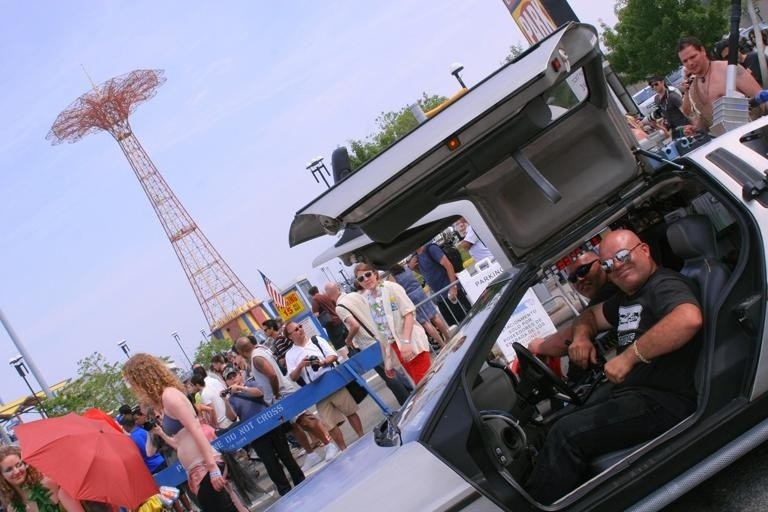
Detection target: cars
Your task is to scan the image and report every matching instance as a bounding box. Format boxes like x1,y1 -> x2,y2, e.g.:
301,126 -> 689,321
627,22 -> 768,114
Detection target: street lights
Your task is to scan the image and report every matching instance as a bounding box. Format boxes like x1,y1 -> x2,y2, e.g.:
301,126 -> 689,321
7,355 -> 49,420
169,326 -> 210,367
114,338 -> 133,357
304,156 -> 332,189
316,259 -> 352,288
445,63 -> 468,88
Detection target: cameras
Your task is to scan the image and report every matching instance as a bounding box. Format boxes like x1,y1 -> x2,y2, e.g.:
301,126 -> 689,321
309,356 -> 320,371
143,419 -> 161,432
641,108 -> 663,125
220,389 -> 231,398
686,77 -> 695,85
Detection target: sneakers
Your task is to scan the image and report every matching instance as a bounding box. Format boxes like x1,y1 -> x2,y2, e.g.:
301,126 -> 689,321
300,452 -> 322,472
325,443 -> 338,461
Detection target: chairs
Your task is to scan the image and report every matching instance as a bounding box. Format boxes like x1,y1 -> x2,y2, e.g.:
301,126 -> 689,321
581,211 -> 730,472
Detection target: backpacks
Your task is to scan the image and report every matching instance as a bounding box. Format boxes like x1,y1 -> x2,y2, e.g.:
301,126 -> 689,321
426,243 -> 463,273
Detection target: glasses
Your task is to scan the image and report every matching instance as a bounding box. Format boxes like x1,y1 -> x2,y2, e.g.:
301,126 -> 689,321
599,243 -> 640,275
290,325 -> 302,333
568,258 -> 599,283
356,272 -> 371,283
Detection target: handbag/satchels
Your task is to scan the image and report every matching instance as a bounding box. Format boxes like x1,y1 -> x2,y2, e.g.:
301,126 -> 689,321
344,343 -> 361,358
317,311 -> 333,329
346,380 -> 369,403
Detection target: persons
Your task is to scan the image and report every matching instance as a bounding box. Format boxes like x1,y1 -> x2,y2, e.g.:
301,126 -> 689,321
0,10 -> 767,511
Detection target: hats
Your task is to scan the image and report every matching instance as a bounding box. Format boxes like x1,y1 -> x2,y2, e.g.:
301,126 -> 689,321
223,367 -> 239,380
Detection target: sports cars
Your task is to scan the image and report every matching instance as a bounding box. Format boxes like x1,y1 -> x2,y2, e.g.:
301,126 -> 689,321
255,21 -> 767,512
